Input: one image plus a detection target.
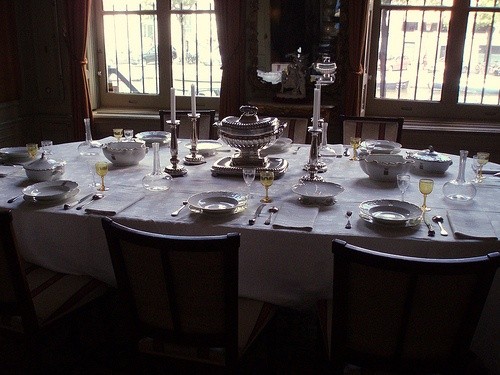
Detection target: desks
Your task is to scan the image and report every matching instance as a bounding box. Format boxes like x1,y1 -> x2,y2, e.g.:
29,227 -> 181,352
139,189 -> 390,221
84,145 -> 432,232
0,142 -> 500,375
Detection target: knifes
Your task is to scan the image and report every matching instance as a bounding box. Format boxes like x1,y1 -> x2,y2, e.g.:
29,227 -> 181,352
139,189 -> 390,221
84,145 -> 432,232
248,204 -> 266,225
64,193 -> 93,209
424,215 -> 435,234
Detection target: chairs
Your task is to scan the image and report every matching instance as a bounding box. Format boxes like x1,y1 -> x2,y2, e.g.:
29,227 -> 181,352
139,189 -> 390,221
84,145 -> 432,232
335,115 -> 406,145
158,109 -> 215,139
259,111 -> 312,144
0,207 -> 125,375
100,216 -> 290,375
325,238 -> 500,375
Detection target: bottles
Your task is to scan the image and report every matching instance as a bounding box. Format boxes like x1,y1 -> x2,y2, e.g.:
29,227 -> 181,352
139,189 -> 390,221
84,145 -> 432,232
142,143 -> 173,192
318,123 -> 335,164
443,150 -> 477,202
77,118 -> 101,159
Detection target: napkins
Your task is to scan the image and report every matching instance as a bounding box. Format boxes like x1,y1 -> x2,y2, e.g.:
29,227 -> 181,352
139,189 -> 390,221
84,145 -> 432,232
448,210 -> 499,240
472,164 -> 500,174
84,190 -> 145,215
0,165 -> 23,177
272,201 -> 321,229
320,143 -> 344,156
91,135 -> 118,147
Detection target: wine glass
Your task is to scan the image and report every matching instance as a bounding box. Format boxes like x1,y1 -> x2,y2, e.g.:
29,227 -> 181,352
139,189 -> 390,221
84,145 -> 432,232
243,167 -> 274,203
87,160 -> 110,191
113,129 -> 133,142
26,140 -> 53,161
397,175 -> 410,202
471,152 -> 489,183
419,179 -> 434,211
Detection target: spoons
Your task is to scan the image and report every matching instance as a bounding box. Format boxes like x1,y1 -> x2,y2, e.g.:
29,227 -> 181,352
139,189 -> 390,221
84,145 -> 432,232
76,193 -> 104,211
432,215 -> 448,236
264,206 -> 278,224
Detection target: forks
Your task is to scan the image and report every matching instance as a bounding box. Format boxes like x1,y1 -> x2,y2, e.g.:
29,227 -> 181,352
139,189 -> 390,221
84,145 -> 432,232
344,210 -> 352,228
170,199 -> 188,216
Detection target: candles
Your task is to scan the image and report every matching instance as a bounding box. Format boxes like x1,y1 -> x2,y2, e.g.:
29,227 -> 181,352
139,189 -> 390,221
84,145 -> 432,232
191,84 -> 196,115
170,86 -> 176,123
313,79 -> 322,129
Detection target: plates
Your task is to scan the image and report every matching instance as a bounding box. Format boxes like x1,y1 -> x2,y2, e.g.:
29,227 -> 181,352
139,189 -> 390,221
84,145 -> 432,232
184,140 -> 222,154
135,131 -> 170,144
21,180 -> 80,204
188,190 -> 248,217
359,199 -> 423,228
261,138 -> 401,155
0,147 -> 39,158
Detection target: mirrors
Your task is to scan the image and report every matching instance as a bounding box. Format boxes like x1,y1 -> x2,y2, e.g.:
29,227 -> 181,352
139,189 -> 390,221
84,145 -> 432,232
242,0 -> 363,95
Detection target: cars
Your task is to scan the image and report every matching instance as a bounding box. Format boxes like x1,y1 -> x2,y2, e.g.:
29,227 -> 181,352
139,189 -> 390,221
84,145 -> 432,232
375,56 -> 500,94
140,43 -> 178,64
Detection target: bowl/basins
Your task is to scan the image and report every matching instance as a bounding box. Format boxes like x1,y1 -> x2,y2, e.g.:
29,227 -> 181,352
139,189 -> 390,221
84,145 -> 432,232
102,141 -> 146,167
359,146 -> 453,183
291,182 -> 344,203
23,151 -> 66,182
